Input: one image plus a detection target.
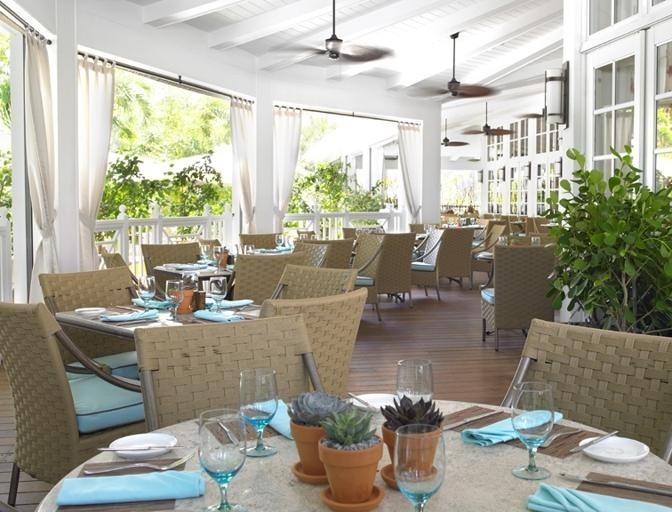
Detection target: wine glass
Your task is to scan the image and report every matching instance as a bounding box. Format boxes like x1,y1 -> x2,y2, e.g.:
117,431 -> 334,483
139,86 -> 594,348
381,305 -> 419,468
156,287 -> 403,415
209,276 -> 228,313
375,227 -> 385,234
311,235 -> 321,240
275,233 -> 284,248
137,275 -> 157,313
392,422 -> 445,512
395,356 -> 434,409
299,234 -> 309,240
165,279 -> 184,323
286,234 -> 295,253
238,368 -> 279,459
181,271 -> 200,292
198,407 -> 247,512
424,224 -> 438,233
245,243 -> 256,256
470,218 -> 475,226
530,236 -> 541,245
355,228 -> 363,237
498,236 -> 508,245
198,244 -> 211,269
213,246 -> 224,275
511,381 -> 555,479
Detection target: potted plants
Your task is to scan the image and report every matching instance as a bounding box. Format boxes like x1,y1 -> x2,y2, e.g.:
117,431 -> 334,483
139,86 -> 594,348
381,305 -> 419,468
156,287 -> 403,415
286,391 -> 344,484
377,394 -> 446,492
316,405 -> 386,512
538,140 -> 672,340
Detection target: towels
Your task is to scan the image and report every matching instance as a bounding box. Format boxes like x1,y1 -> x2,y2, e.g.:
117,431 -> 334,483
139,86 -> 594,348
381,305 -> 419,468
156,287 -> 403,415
247,400 -> 296,441
461,410 -> 563,447
526,483 -> 672,512
55,469 -> 207,505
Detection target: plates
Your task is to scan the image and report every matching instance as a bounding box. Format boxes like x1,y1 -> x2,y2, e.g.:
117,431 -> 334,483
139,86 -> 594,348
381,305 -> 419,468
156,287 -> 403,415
109,432 -> 179,459
319,486 -> 385,512
350,394 -> 398,414
290,463 -> 329,484
73,307 -> 105,317
578,436 -> 651,464
381,463 -> 439,490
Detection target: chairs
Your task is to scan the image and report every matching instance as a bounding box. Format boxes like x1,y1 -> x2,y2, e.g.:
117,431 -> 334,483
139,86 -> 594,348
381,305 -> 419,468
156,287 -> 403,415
500,318 -> 672,461
0,303 -> 145,507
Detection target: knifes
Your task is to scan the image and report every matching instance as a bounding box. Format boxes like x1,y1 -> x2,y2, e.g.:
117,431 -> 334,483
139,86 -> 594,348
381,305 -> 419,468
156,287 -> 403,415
94,445 -> 183,452
441,409 -> 504,430
216,419 -> 240,444
347,392 -> 378,412
558,472 -> 672,497
570,430 -> 620,453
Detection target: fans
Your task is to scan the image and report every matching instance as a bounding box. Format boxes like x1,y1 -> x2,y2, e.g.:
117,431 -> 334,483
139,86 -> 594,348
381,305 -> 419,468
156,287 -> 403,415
460,102 -> 516,136
264,0 -> 391,68
407,30 -> 500,103
440,103 -> 469,147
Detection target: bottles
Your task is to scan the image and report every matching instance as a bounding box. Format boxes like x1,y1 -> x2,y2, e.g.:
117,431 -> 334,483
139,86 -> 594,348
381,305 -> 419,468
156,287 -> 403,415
227,255 -> 234,265
194,290 -> 206,310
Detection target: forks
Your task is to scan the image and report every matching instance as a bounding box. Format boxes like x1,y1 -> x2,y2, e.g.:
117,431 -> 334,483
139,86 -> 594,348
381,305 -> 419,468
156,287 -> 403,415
83,451 -> 196,476
